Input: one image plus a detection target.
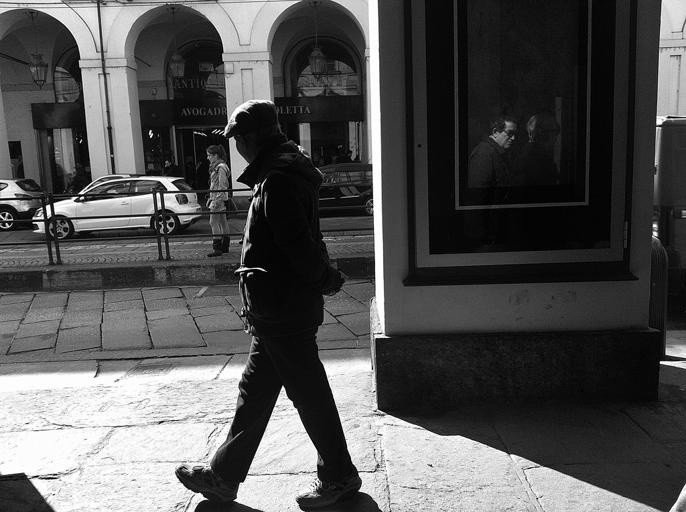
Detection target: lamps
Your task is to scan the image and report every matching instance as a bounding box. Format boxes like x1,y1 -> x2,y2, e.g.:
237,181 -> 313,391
167,5 -> 185,82
308,1 -> 326,82
25,10 -> 48,89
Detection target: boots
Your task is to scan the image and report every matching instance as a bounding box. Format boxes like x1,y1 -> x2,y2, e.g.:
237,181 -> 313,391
223,237 -> 230,253
207,239 -> 223,257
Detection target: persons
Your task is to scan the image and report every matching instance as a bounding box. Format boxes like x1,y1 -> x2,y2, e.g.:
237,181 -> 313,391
511,111 -> 561,185
468,110 -> 519,189
175,99 -> 362,510
164,160 -> 179,177
206,144 -> 231,257
70,167 -> 91,196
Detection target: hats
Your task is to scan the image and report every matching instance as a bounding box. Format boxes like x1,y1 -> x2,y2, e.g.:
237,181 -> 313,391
224,99 -> 279,138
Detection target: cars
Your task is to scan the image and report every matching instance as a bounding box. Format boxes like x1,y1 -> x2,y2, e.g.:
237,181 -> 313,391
70,174 -> 140,195
30,173 -> 205,241
318,160 -> 374,218
0,177 -> 49,232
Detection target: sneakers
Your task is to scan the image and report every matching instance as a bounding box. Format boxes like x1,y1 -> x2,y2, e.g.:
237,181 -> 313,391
175,463 -> 239,508
296,466 -> 362,508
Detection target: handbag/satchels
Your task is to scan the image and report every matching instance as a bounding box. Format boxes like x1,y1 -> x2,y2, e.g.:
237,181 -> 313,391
224,198 -> 239,211
